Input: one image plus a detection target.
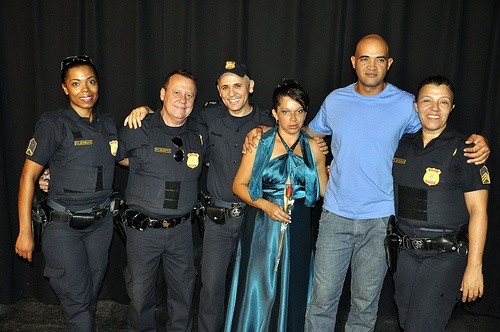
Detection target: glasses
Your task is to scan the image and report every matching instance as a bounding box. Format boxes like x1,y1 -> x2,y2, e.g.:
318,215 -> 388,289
173,136 -> 185,162
60,55 -> 94,71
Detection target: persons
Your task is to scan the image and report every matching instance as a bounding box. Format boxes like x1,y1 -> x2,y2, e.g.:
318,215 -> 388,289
240,35 -> 491,332
16,55 -> 129,332
224,77 -> 328,332
124,59 -> 329,332
324,73 -> 492,332
39,68 -> 208,332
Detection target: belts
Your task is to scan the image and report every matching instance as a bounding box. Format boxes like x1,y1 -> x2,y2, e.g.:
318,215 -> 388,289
394,227 -> 467,251
123,206 -> 192,229
43,206 -> 110,222
205,207 -> 245,219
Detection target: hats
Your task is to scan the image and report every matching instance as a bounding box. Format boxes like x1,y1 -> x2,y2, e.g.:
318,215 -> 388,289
216,57 -> 249,78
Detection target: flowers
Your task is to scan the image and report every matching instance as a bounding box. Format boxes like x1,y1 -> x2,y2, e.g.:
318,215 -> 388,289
274,184 -> 295,271
